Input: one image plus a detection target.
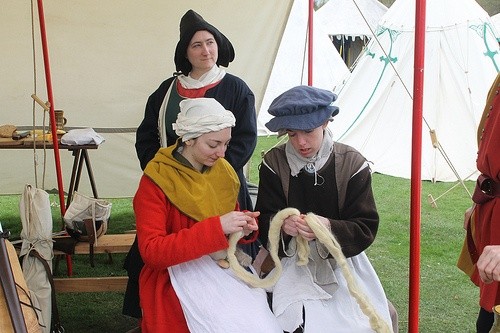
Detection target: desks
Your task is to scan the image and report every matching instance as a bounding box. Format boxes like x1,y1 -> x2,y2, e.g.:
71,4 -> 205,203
0,130 -> 113,267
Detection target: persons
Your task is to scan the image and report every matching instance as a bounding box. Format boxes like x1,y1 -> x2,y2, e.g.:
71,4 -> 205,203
121,10 -> 257,333
253,86 -> 393,333
132,97 -> 283,333
458,75 -> 500,333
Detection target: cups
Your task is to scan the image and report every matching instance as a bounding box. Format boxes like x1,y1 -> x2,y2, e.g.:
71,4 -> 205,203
49,110 -> 67,131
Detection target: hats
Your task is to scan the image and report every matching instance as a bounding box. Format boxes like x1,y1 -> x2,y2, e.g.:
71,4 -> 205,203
174,9 -> 235,76
265,85 -> 339,132
175,97 -> 236,142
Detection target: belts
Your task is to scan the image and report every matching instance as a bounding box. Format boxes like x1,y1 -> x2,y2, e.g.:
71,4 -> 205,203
464,174 -> 500,266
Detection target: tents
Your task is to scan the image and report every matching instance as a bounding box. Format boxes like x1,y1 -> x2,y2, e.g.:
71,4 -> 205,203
315,0 -> 389,71
256,0 -> 350,136
328,0 -> 500,182
